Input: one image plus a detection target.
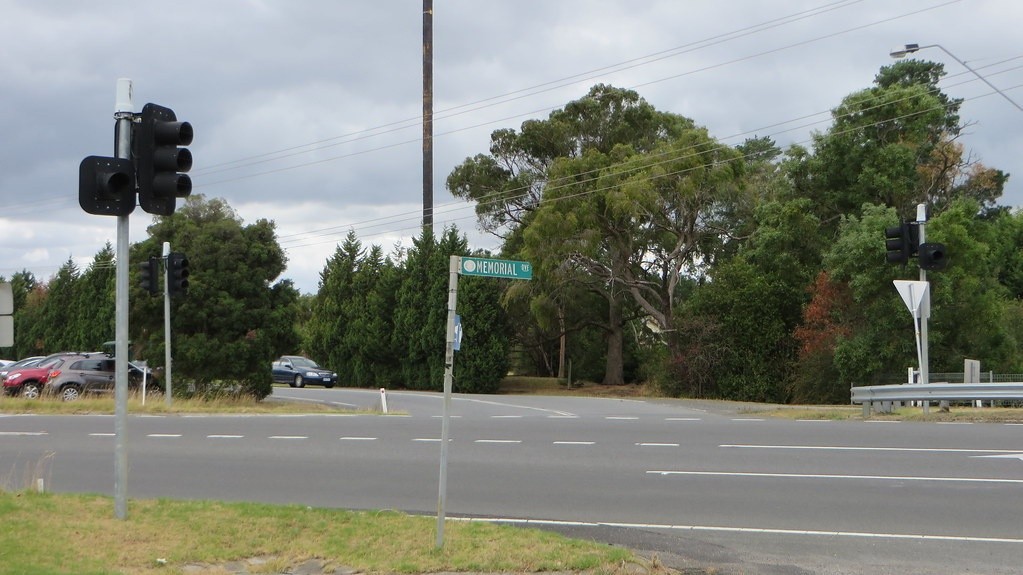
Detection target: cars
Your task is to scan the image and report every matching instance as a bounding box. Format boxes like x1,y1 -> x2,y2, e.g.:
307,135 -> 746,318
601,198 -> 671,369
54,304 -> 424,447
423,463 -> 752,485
269,355 -> 340,388
0,351 -> 164,403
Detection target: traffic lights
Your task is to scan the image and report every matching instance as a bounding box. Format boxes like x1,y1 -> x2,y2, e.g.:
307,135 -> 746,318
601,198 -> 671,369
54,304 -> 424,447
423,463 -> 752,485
885,216 -> 912,266
168,253 -> 190,297
139,256 -> 159,297
918,242 -> 947,270
139,103 -> 193,201
78,155 -> 137,216
